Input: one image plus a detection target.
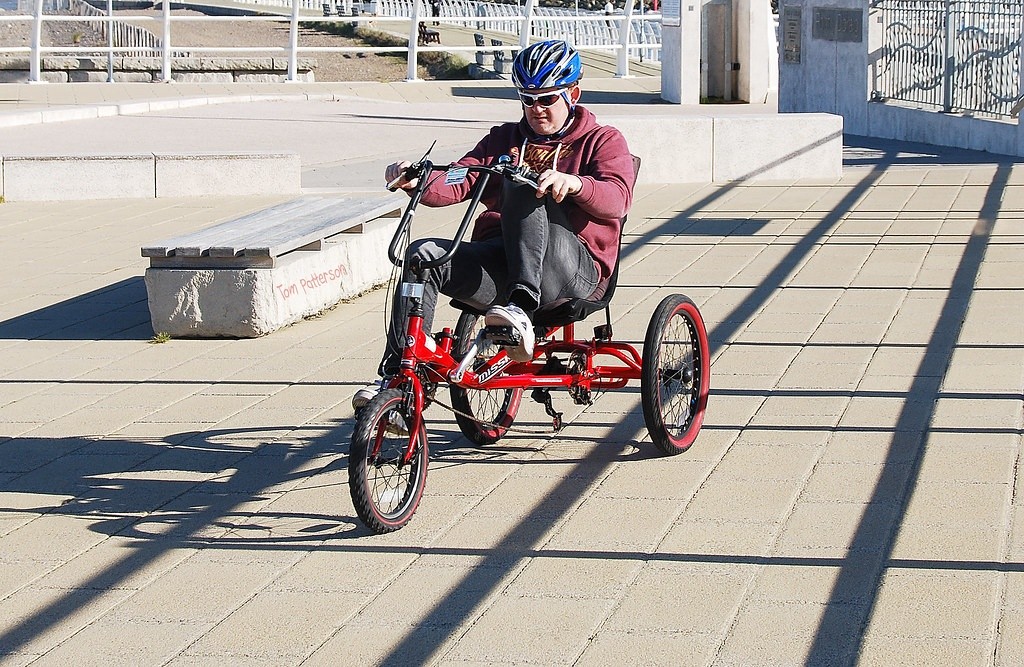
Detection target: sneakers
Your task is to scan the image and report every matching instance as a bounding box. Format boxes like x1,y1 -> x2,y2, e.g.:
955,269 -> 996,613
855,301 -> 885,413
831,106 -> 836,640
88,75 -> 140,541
353,384 -> 409,435
485,304 -> 534,362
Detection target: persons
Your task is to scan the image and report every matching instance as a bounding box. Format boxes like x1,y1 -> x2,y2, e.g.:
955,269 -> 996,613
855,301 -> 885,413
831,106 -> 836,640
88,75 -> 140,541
428,0 -> 444,27
605,0 -> 613,41
352,37 -> 636,436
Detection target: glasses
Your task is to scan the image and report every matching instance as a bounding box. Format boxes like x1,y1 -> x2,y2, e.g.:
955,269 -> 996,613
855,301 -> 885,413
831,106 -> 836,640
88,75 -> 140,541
518,87 -> 569,107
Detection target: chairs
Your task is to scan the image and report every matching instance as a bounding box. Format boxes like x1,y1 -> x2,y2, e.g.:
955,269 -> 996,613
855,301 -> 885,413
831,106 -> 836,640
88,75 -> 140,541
474,33 -> 504,65
322,3 -> 336,17
511,43 -> 518,60
449,152 -> 642,322
490,39 -> 513,74
351,7 -> 368,26
337,6 -> 352,17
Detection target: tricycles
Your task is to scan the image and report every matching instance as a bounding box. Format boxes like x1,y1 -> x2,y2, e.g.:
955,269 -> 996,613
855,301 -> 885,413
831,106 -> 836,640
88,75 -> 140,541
348,140 -> 711,534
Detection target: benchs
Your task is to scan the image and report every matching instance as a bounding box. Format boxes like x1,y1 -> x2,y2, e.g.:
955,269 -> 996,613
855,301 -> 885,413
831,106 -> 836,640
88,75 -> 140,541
140,193 -> 410,339
419,20 -> 441,45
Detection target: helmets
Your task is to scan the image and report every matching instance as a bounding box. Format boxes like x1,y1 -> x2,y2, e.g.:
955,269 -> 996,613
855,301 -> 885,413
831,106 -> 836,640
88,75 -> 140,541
513,40 -> 582,89
